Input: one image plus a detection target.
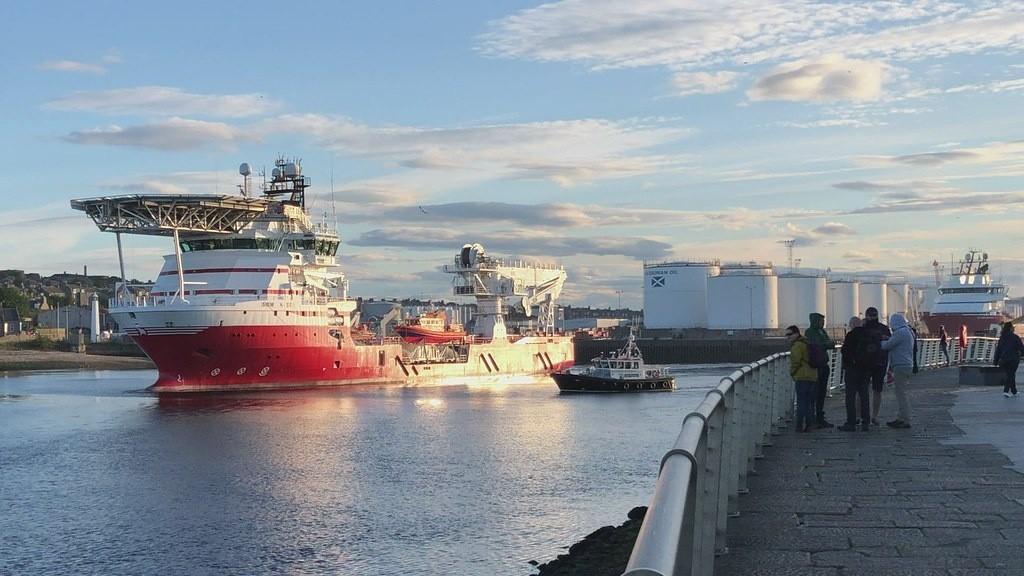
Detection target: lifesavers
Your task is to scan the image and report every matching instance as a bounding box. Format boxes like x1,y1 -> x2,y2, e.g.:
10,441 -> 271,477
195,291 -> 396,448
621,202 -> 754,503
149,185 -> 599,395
959,324 -> 967,347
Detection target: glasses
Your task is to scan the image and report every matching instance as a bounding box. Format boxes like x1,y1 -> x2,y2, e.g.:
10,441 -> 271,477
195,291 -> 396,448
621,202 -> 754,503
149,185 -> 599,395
786,331 -> 797,336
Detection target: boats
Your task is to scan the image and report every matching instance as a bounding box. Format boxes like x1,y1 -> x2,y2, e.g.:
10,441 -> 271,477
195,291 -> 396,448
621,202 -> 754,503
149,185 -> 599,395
69,153 -> 578,394
549,312 -> 678,393
917,249 -> 1012,344
393,309 -> 469,347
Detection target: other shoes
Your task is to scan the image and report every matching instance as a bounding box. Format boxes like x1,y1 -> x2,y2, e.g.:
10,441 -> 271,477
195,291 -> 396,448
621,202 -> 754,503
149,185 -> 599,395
1002,392 -> 1011,397
861,421 -> 870,431
806,420 -> 823,430
1012,392 -> 1021,397
886,420 -> 903,428
870,418 -> 880,426
894,422 -> 913,430
837,419 -> 856,431
856,418 -> 863,426
796,425 -> 811,433
817,419 -> 834,427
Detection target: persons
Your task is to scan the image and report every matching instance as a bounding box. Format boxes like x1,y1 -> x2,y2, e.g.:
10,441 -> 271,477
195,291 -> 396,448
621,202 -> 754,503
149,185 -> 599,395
786,307 -> 917,433
938,324 -> 951,367
993,322 -> 1024,398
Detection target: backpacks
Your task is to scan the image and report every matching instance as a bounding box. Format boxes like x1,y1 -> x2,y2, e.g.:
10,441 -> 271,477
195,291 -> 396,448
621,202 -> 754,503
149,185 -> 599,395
798,338 -> 825,369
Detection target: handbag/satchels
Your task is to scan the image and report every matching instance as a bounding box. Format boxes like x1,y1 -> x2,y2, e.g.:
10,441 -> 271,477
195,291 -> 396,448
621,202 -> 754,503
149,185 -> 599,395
844,344 -> 865,376
